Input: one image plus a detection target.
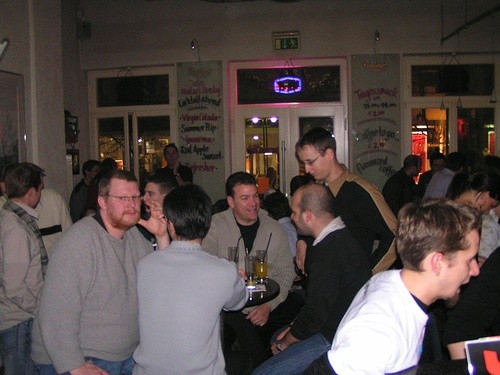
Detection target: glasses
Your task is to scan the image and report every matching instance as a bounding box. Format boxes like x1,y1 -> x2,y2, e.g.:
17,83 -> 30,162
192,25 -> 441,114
300,154 -> 320,168
108,195 -> 141,202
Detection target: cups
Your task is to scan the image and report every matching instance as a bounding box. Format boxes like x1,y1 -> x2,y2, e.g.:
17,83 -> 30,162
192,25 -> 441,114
228,247 -> 240,265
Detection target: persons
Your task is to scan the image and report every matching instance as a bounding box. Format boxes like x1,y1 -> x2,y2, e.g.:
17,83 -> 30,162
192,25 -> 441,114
0,126 -> 500,310
29,169 -> 171,375
441,245 -> 500,360
131,185 -> 249,375
201,172 -> 305,375
302,196 -> 483,375
250,180 -> 373,375
0,162 -> 49,375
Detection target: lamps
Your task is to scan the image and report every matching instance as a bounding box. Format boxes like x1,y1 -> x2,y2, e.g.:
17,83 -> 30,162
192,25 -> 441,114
374,30 -> 383,54
191,39 -> 200,63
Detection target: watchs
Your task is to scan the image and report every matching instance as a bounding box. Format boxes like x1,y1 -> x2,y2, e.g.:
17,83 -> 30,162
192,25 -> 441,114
276,340 -> 281,352
173,173 -> 180,178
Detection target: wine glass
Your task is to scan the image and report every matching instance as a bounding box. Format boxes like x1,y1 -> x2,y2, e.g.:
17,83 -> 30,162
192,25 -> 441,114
244,249 -> 268,282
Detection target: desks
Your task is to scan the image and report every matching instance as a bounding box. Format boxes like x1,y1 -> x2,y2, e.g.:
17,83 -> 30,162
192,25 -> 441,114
242,277 -> 281,307
